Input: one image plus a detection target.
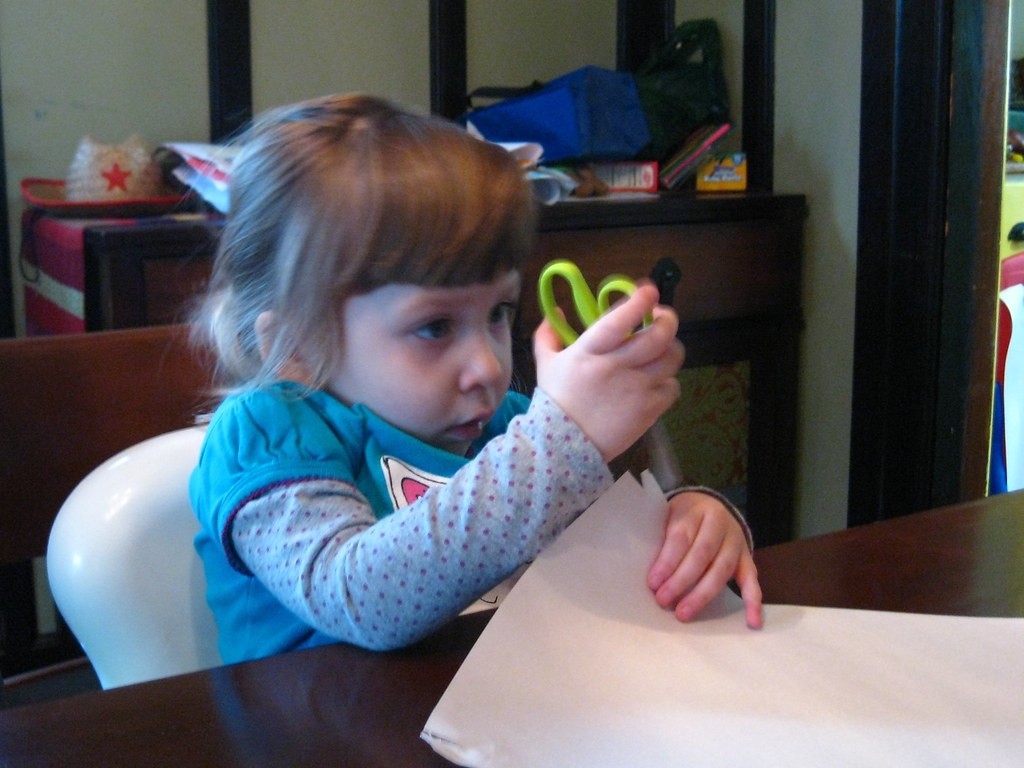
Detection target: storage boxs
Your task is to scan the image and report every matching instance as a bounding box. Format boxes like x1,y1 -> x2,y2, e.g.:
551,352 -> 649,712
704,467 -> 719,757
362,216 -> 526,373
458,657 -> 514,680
588,162 -> 658,192
696,151 -> 747,191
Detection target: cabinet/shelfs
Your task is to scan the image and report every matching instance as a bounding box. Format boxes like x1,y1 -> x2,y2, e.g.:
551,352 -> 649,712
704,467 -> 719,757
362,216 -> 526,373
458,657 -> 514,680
21,186 -> 810,552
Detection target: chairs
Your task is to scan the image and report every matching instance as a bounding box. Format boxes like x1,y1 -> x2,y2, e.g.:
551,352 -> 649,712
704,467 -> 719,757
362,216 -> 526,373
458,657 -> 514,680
46,423 -> 224,691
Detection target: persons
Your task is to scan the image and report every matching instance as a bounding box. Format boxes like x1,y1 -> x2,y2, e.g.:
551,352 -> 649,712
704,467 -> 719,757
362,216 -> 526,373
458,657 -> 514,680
988,222 -> 1024,497
191,94 -> 766,666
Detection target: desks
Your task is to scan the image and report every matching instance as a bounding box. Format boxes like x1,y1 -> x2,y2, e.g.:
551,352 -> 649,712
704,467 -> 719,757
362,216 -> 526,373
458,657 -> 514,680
0,490 -> 1022,768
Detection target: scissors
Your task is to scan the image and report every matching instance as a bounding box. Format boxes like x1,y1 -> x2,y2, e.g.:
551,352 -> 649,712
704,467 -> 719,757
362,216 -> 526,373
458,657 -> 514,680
538,259 -> 681,500
